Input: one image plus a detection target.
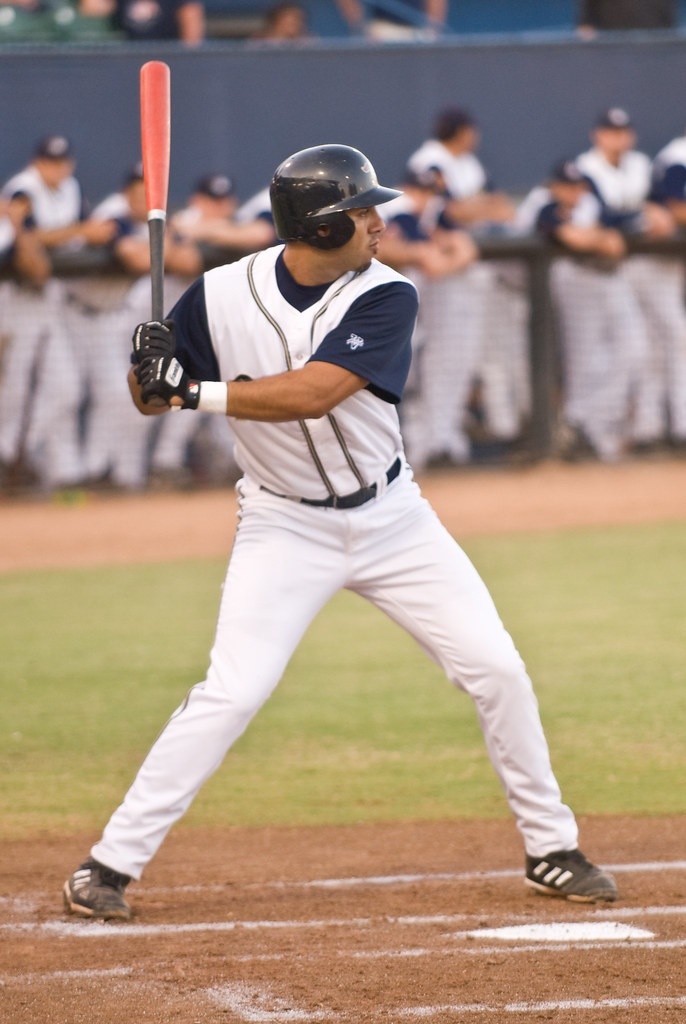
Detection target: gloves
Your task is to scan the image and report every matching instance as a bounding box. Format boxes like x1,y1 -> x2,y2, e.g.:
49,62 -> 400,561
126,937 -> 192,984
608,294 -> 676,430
134,355 -> 200,410
129,320 -> 174,364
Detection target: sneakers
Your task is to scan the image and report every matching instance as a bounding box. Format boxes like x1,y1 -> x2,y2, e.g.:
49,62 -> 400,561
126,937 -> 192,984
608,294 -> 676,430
525,848 -> 616,902
63,855 -> 131,920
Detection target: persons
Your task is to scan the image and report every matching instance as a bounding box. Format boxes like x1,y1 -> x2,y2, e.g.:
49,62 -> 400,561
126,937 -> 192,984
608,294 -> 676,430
575,0 -> 675,39
0,135 -> 286,492
373,116 -> 685,477
63,146 -> 622,917
7,0 -> 447,50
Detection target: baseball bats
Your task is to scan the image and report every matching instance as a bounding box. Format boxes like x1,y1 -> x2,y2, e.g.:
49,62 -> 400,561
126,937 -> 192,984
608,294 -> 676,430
137,61 -> 171,412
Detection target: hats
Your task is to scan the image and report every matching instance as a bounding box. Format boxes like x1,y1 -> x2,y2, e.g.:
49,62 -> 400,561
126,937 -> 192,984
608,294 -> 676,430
37,137 -> 72,158
196,174 -> 235,198
598,110 -> 636,130
552,162 -> 584,183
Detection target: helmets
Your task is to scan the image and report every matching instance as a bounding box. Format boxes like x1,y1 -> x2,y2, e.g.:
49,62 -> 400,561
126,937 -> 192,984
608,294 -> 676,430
269,143 -> 404,249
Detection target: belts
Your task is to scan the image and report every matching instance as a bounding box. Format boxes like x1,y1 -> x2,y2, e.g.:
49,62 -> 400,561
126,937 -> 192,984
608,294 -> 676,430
262,458 -> 401,510
